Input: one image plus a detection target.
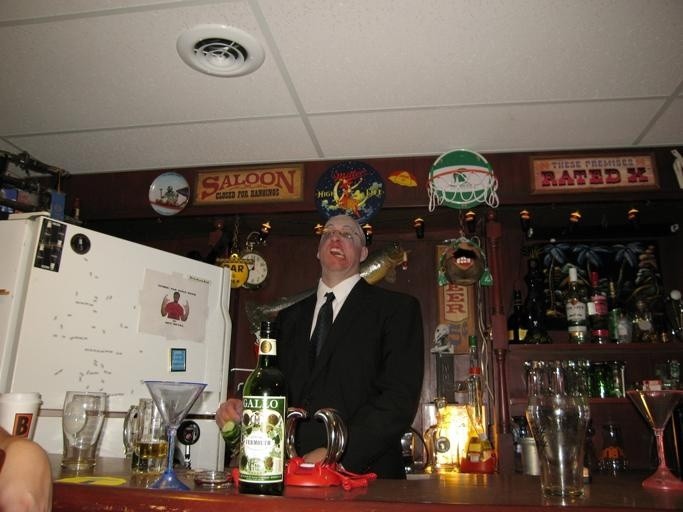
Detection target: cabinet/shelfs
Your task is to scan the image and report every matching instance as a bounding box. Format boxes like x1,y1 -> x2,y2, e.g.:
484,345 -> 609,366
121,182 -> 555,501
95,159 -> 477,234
0,145 -> 683,471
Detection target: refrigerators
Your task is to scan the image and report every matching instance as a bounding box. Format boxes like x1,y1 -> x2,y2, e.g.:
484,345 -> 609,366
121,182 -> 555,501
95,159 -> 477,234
0,209 -> 233,472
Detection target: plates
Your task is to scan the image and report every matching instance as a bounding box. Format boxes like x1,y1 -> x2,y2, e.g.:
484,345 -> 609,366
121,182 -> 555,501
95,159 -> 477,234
147,171 -> 192,217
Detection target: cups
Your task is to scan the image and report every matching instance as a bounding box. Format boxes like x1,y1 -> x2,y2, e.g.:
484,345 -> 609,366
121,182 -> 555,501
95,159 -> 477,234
58,390 -> 106,473
0,391 -> 43,442
120,399 -> 169,473
518,437 -> 539,478
523,364 -> 591,499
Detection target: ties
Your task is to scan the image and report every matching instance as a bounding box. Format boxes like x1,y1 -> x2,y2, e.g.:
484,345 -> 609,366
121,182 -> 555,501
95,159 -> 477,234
309,292 -> 335,368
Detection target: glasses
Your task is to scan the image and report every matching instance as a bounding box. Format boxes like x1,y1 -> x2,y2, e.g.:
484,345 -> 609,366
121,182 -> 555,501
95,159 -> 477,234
322,229 -> 365,247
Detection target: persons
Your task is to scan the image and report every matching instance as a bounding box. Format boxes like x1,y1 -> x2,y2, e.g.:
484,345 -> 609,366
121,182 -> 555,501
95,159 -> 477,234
214,215 -> 425,481
0,423 -> 53,512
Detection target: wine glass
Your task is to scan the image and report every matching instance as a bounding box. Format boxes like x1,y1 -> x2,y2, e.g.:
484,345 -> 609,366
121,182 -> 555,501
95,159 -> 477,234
143,378 -> 207,492
625,389 -> 682,492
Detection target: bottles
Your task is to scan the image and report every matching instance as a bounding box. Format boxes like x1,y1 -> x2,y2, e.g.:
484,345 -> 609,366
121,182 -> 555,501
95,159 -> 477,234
466,335 -> 483,406
601,422 -> 625,473
526,359 -> 624,401
560,266 -> 653,345
238,321 -> 290,495
506,287 -> 527,342
